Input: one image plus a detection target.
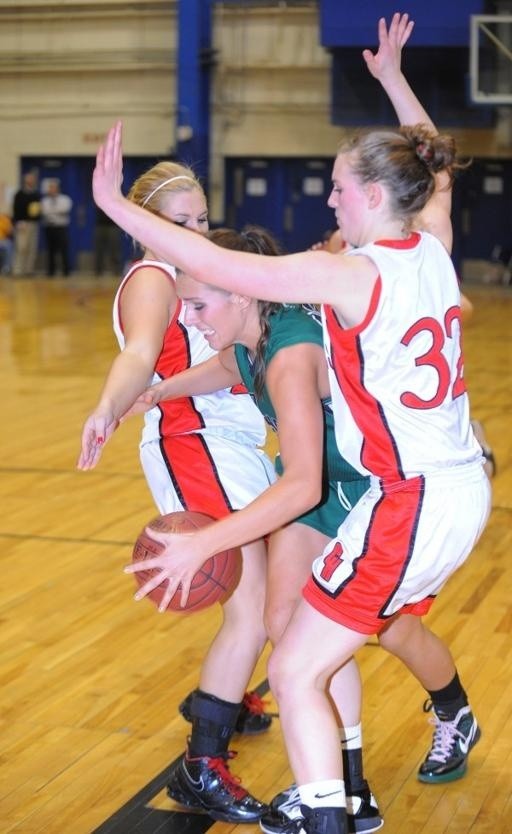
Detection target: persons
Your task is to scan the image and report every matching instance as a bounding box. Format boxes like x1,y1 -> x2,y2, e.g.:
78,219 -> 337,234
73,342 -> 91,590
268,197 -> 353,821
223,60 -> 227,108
120,228 -> 385,834
91,11 -> 493,834
40,181 -> 73,279
76,161 -> 279,824
94,184 -> 125,276
12,172 -> 42,278
0,212 -> 15,276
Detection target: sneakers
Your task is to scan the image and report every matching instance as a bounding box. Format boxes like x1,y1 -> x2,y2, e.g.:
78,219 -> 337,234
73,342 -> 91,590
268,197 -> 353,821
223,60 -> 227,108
179,688 -> 271,732
418,705 -> 481,783
259,783 -> 384,833
166,736 -> 269,824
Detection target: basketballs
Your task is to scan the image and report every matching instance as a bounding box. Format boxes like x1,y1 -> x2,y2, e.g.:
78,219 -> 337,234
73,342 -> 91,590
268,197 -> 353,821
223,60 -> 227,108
132,511 -> 239,614
